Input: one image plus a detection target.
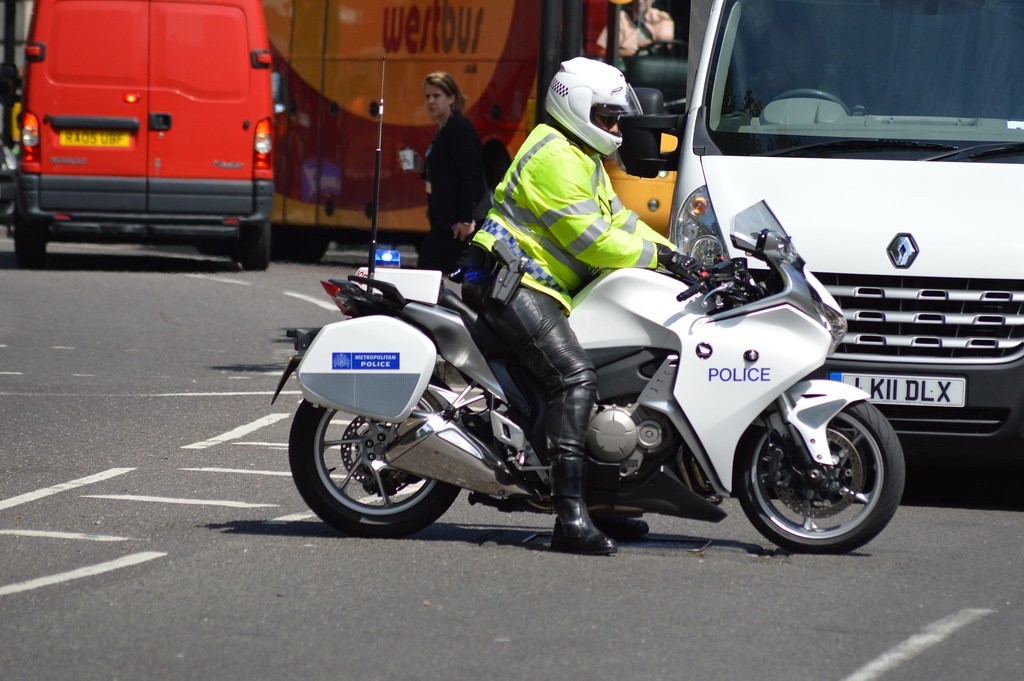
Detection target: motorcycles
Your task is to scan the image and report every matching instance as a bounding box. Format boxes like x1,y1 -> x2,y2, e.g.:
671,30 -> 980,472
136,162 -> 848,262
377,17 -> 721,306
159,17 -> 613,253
272,196 -> 910,534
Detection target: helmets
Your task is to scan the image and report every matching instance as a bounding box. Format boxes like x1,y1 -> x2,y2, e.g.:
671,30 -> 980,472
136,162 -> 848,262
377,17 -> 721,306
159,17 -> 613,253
544,56 -> 644,155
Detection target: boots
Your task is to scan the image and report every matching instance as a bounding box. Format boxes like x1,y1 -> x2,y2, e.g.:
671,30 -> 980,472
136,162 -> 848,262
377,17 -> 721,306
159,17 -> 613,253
589,513 -> 649,540
551,457 -> 618,555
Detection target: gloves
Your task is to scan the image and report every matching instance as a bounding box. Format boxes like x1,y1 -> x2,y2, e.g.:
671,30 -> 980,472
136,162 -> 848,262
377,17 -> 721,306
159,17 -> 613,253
655,242 -> 709,282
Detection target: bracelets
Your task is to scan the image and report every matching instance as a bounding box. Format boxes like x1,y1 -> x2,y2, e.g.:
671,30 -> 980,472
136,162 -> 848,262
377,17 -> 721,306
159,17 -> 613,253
461,220 -> 473,225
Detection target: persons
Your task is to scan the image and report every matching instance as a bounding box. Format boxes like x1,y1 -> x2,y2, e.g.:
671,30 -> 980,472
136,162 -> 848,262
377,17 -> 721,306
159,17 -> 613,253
597,0 -> 675,70
400,72 -> 488,276
462,57 -> 716,556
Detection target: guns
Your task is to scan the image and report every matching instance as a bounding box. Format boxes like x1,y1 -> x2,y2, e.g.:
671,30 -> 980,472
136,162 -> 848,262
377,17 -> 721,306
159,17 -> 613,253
489,239 -> 528,306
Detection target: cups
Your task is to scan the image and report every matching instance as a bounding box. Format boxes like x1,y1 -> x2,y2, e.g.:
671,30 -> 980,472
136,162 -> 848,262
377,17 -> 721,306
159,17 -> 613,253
399,149 -> 414,170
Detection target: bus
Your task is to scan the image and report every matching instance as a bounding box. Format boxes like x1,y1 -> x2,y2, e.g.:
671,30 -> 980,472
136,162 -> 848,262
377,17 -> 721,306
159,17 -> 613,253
273,0 -> 714,267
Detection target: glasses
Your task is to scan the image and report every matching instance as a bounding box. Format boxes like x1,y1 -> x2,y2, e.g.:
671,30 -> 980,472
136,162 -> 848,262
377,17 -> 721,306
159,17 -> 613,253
599,115 -> 623,130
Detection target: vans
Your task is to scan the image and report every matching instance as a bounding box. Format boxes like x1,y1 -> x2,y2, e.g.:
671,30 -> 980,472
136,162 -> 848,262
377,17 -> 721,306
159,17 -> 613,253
621,0 -> 1024,512
0,0 -> 275,271
